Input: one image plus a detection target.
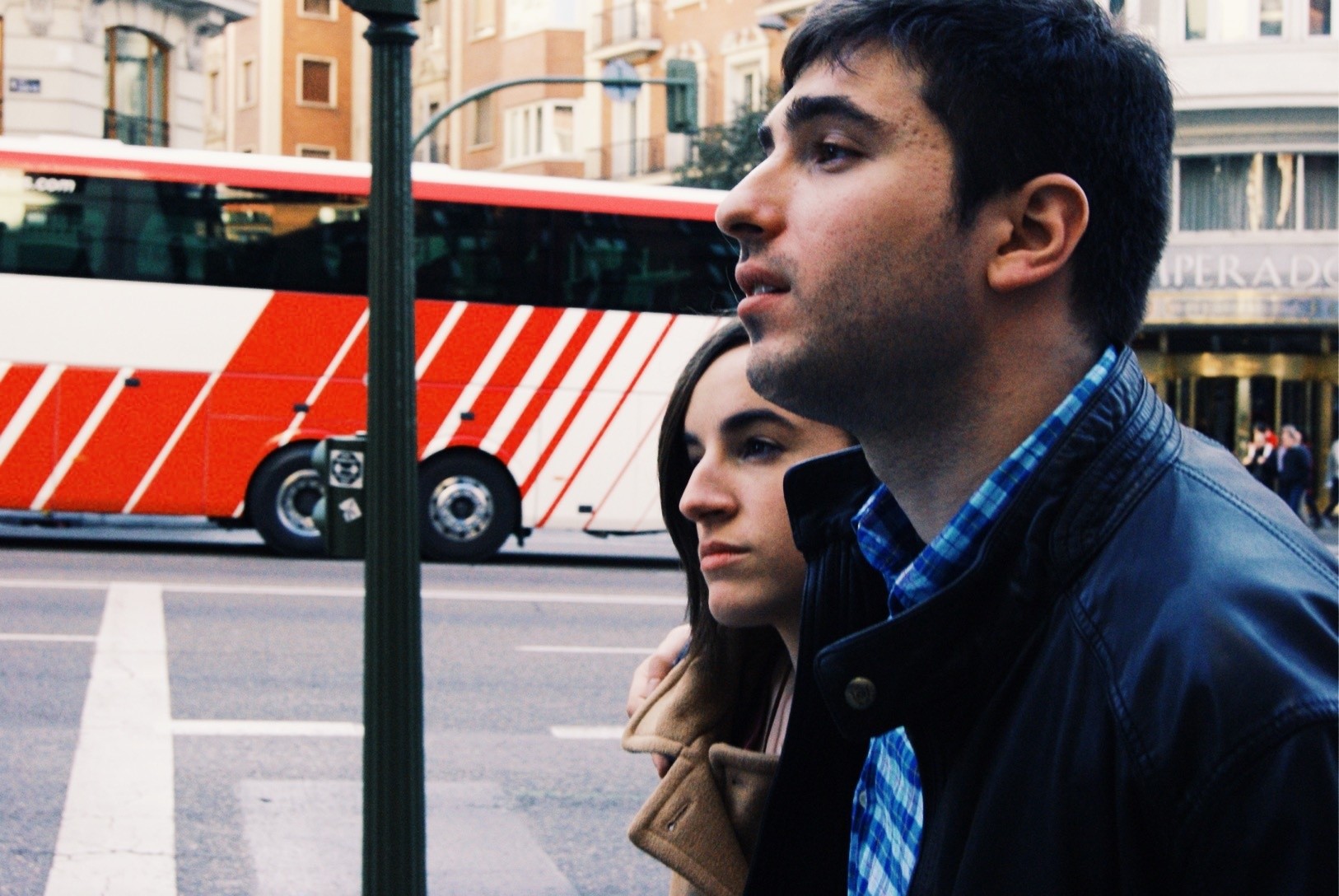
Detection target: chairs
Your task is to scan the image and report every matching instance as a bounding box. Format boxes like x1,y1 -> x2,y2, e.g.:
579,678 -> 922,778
10,229 -> 736,314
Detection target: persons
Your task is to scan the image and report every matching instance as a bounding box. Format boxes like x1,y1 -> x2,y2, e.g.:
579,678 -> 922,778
1323,438 -> 1339,523
1243,423 -> 1274,489
1265,427 -> 1277,449
621,314 -> 862,896
1272,423 -> 1312,524
1298,430 -> 1323,531
709,0 -> 1339,896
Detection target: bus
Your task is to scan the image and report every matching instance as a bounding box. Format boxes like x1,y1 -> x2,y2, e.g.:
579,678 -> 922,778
0,138 -> 741,568
